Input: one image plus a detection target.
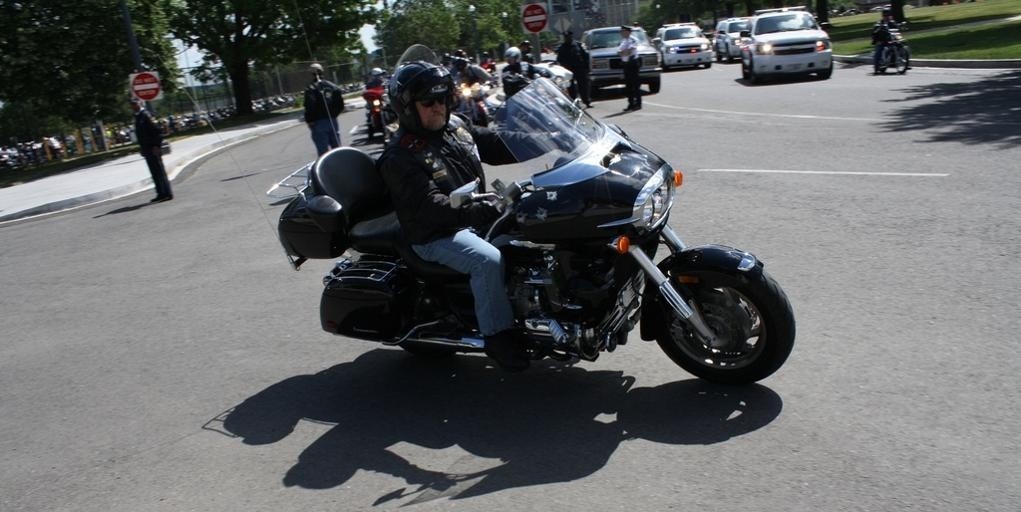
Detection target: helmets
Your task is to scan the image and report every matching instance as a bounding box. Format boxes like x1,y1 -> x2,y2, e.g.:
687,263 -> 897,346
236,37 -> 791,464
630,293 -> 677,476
504,47 -> 522,62
388,60 -> 461,135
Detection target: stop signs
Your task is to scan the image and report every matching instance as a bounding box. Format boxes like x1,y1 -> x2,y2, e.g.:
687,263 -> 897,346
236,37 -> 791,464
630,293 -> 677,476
518,2 -> 551,35
128,71 -> 164,104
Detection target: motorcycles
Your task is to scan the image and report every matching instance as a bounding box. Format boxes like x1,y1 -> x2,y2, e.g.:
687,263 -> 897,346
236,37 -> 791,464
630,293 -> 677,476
265,77 -> 795,387
865,19 -> 911,75
355,47 -> 581,129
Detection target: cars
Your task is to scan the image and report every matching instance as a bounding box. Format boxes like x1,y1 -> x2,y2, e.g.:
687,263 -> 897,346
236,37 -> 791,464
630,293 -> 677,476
740,6 -> 834,84
824,3 -> 893,17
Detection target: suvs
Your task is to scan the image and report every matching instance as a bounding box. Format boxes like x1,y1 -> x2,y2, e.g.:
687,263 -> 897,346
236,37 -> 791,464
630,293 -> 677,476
577,25 -> 661,96
651,22 -> 713,71
712,17 -> 752,59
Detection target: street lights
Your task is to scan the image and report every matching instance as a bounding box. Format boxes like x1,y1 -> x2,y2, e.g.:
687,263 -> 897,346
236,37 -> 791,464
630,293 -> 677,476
466,2 -> 483,57
499,12 -> 514,52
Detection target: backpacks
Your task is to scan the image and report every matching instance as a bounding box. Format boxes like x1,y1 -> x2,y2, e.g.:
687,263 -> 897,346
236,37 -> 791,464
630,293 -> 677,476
310,80 -> 344,118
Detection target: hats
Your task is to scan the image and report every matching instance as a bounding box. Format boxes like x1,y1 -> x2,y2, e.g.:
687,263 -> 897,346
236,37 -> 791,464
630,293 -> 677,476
372,68 -> 387,76
620,25 -> 633,31
309,63 -> 326,73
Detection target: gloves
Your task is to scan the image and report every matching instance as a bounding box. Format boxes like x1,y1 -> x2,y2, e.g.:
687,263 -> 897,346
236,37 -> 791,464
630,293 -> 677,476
458,201 -> 502,229
551,134 -> 575,151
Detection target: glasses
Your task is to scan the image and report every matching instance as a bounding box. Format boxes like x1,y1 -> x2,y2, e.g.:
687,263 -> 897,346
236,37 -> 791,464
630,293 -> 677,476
420,96 -> 446,108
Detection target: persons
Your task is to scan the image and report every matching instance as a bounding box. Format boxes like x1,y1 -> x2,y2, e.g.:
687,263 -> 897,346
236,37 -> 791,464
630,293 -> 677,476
556,30 -> 594,108
873,10 -> 906,75
440,40 -> 553,101
616,25 -> 642,111
302,63 -> 346,156
374,57 -> 575,351
363,67 -> 389,129
130,97 -> 173,204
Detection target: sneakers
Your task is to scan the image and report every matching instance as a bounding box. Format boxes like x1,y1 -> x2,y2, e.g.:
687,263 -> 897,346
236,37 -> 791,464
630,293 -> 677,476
484,330 -> 534,371
